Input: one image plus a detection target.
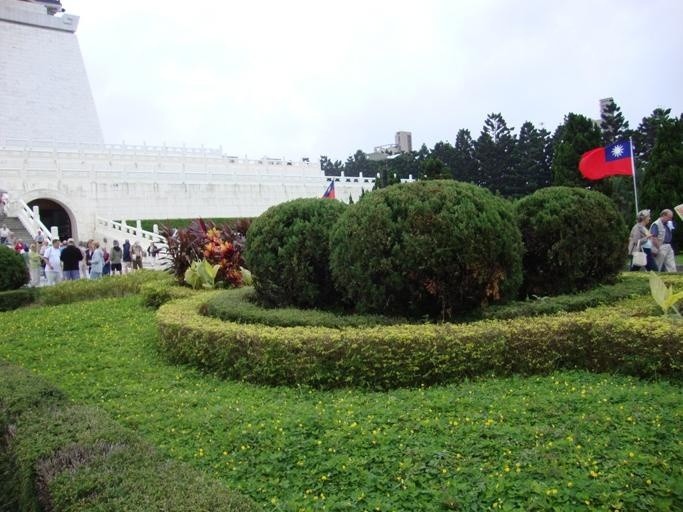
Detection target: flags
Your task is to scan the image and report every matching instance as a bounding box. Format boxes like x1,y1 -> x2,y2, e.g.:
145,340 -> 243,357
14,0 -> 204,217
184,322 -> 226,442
576,138 -> 633,182
319,180 -> 335,200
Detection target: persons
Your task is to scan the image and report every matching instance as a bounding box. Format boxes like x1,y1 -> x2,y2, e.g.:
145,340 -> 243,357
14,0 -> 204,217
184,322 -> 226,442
0,223 -> 157,289
648,208 -> 678,273
627,209 -> 659,273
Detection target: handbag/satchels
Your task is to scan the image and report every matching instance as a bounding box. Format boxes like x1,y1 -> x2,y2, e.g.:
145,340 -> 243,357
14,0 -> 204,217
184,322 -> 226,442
631,240 -> 647,266
131,253 -> 137,261
